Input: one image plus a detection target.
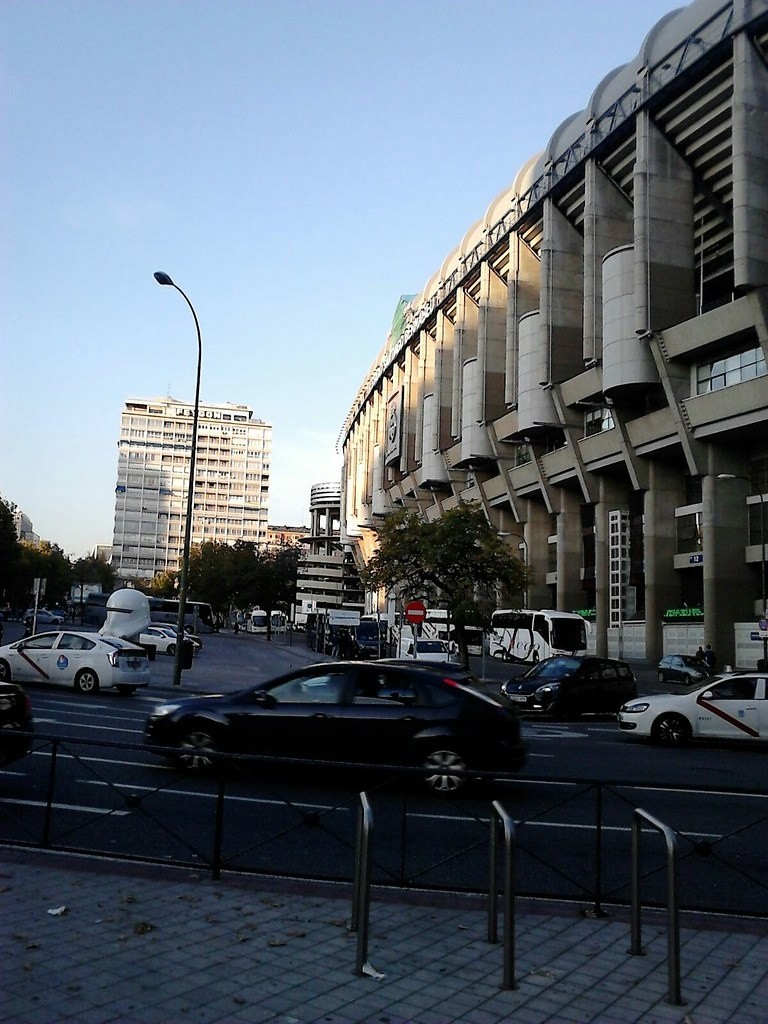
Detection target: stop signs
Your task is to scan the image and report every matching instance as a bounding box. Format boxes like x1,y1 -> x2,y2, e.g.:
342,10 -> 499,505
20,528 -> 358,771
405,601 -> 426,623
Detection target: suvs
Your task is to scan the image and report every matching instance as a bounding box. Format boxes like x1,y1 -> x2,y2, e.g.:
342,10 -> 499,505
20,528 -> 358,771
499,654 -> 639,721
615,670 -> 768,748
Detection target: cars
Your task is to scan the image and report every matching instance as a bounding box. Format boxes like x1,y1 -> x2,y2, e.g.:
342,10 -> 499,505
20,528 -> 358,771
0,631 -> 151,695
23,610 -> 65,625
150,622 -> 202,649
292,622 -> 306,631
139,627 -> 201,657
290,658 -> 485,698
396,637 -> 452,663
656,655 -> 714,686
0,603 -> 22,613
142,662 -> 529,798
307,628 -> 359,657
0,681 -> 34,766
355,621 -> 390,656
6,615 -> 22,622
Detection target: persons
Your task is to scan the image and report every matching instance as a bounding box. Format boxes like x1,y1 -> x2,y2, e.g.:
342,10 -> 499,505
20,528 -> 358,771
705,645 -> 714,667
233,622 -> 239,635
214,618 -> 220,633
696,647 -> 705,661
23,610 -> 37,638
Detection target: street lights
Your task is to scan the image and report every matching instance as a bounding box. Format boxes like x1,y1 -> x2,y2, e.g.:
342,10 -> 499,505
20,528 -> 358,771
154,271 -> 202,685
718,474 -> 767,672
497,532 -> 528,610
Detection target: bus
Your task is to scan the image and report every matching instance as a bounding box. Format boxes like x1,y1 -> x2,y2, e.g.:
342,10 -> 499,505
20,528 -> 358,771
489,609 -> 591,667
361,609 -> 483,657
86,593 -> 215,635
270,610 -> 288,632
236,610 -> 268,632
